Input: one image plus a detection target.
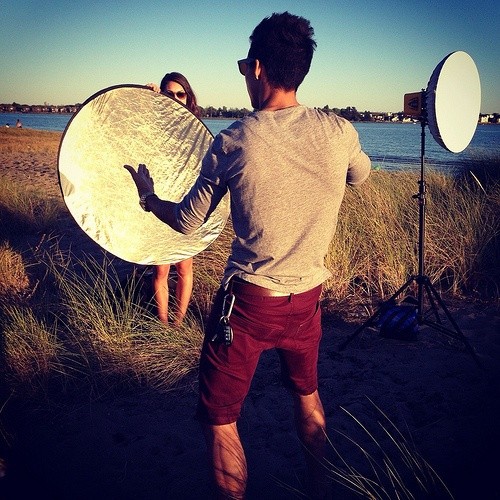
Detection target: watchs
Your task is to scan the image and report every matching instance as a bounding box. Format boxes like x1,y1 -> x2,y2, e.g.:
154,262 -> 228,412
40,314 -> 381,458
140,192 -> 156,212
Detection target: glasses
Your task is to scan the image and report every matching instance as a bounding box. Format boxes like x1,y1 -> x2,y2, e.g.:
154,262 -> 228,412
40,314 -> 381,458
237,57 -> 256,76
165,89 -> 187,100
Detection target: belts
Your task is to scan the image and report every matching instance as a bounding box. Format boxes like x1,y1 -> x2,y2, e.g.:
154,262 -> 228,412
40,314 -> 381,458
228,280 -> 308,298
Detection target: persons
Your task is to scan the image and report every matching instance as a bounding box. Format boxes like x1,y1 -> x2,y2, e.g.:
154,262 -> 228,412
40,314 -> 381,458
16,119 -> 23,129
119,10 -> 374,500
144,71 -> 203,326
6,123 -> 10,129
